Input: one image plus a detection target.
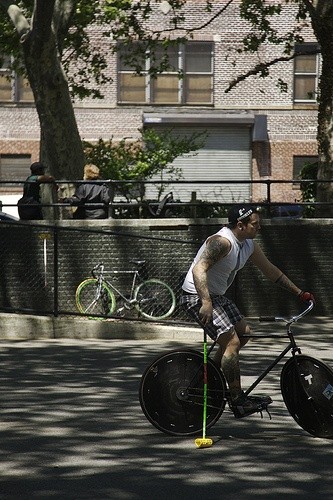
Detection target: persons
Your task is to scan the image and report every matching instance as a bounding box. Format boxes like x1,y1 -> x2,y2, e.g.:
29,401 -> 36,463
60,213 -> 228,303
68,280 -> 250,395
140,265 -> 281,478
179,203 -> 317,418
58,164 -> 110,219
18,162 -> 55,220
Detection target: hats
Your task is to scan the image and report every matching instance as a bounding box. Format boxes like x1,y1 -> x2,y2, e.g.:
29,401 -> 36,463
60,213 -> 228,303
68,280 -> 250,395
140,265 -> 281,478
30,162 -> 48,171
228,204 -> 255,225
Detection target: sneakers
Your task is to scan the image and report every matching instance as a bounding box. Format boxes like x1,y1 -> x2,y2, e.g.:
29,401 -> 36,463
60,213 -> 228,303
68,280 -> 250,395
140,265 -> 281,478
228,391 -> 271,419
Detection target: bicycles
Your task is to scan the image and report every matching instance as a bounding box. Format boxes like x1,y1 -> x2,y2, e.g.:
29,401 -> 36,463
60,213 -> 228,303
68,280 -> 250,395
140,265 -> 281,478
138,300 -> 333,437
76,261 -> 177,321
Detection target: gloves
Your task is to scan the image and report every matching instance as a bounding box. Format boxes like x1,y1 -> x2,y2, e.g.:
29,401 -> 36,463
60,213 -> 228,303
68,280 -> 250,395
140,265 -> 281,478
297,290 -> 316,307
